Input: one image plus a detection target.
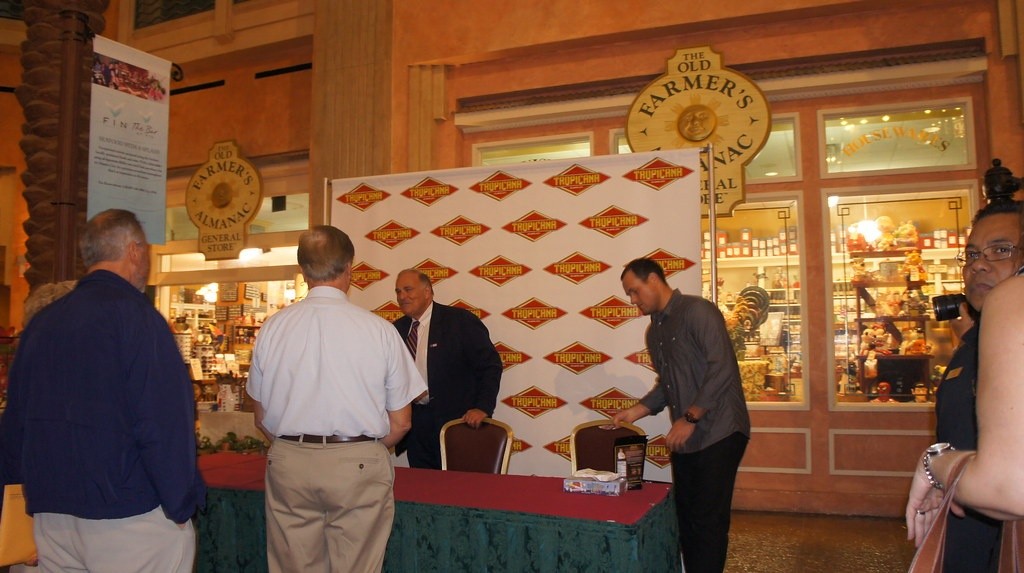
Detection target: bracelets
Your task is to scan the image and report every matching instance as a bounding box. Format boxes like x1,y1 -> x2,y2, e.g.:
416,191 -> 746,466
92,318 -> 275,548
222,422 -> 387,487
684,413 -> 698,423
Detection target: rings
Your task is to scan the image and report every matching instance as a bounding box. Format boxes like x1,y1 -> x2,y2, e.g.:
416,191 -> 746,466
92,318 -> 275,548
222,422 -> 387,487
916,512 -> 925,514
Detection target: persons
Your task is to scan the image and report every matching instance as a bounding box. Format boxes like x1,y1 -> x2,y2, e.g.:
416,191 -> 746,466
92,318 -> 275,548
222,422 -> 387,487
906,197 -> 1024,573
870,382 -> 894,403
910,383 -> 931,403
392,269 -> 502,468
0,209 -> 205,573
245,225 -> 425,573
603,258 -> 751,573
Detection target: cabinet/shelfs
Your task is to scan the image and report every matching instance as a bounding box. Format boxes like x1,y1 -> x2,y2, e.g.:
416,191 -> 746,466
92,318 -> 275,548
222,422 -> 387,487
170,282 -> 267,380
702,223 -> 965,400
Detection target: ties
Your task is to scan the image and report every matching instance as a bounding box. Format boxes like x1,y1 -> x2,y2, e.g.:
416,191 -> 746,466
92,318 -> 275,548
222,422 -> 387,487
406,321 -> 421,361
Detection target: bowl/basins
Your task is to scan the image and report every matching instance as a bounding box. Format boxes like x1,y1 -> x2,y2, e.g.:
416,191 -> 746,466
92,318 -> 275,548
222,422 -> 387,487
196,402 -> 213,411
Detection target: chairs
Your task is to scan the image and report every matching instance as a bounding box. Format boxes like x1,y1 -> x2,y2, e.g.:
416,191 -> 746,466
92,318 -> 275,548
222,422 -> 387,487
570,419 -> 646,476
441,417 -> 514,475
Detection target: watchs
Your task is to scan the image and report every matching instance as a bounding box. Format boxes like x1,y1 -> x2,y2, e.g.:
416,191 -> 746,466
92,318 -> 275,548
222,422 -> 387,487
924,443 -> 957,489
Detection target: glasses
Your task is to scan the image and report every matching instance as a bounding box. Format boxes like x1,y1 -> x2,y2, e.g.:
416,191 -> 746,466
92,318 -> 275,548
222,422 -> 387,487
956,244 -> 1023,267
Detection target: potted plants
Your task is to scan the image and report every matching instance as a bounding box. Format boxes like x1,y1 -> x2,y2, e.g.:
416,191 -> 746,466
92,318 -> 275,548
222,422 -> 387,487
196,428 -> 264,454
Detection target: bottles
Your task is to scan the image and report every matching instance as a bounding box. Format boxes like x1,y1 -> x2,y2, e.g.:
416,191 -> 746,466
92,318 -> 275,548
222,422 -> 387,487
217,384 -> 235,413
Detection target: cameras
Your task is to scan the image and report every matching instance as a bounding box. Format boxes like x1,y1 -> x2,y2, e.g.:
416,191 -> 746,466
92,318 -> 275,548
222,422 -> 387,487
932,292 -> 981,324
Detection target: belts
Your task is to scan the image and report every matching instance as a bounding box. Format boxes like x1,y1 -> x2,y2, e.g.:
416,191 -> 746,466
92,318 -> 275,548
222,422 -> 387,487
279,435 -> 379,444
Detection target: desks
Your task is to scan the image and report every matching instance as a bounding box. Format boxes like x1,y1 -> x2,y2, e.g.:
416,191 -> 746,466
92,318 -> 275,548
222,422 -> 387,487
192,453 -> 685,573
